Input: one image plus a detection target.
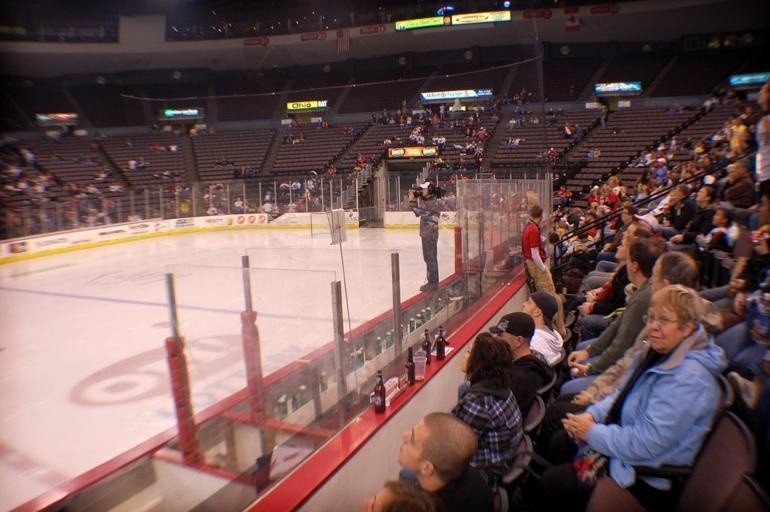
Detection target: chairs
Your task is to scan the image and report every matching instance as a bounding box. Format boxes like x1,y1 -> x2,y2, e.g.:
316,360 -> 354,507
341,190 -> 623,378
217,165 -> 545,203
586,373 -> 770,511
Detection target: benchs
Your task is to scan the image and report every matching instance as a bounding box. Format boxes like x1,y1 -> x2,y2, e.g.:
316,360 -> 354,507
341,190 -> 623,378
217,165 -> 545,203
0,101 -> 761,239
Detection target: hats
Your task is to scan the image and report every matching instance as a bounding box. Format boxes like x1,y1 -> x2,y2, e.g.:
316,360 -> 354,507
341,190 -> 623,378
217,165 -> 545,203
419,181 -> 431,190
488,311 -> 535,338
530,291 -> 559,332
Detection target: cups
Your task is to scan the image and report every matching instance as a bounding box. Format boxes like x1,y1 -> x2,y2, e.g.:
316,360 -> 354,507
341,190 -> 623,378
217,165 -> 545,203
414,350 -> 427,381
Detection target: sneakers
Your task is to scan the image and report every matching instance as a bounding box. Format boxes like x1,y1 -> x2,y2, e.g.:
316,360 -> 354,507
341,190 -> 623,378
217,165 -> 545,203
420,283 -> 439,291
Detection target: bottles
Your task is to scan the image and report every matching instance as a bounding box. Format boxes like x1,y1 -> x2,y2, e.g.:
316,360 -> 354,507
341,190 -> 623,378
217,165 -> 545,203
374,369 -> 386,413
423,330 -> 433,365
403,346 -> 416,386
437,325 -> 445,363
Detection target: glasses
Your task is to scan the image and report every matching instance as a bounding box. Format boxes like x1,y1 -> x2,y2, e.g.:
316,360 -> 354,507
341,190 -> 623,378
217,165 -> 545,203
641,315 -> 684,327
